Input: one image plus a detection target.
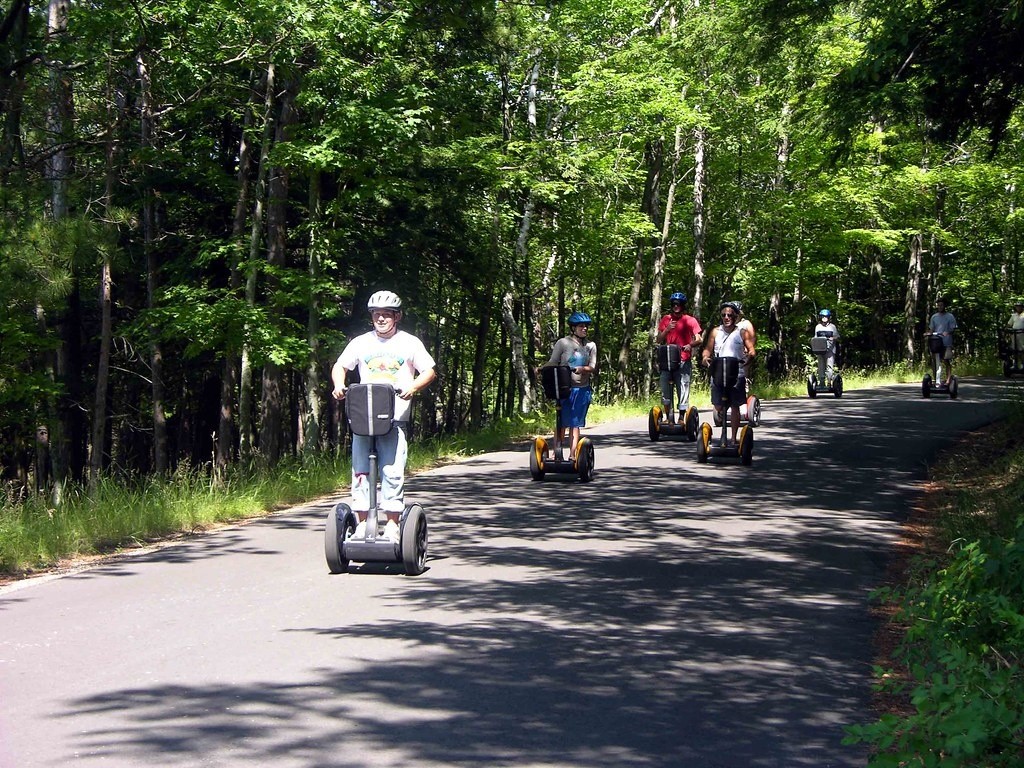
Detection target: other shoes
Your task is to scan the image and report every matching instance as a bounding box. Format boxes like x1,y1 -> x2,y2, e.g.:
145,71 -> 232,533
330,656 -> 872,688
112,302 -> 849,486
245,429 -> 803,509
349,519 -> 367,541
729,439 -> 735,448
825,383 -> 832,390
568,455 -> 576,461
1010,366 -> 1015,369
676,419 -> 685,425
943,382 -> 949,387
815,384 -> 826,391
373,518 -> 401,541
660,419 -> 669,425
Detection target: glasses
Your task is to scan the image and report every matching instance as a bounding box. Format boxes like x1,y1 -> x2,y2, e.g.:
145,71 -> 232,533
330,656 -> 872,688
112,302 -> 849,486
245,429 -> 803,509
671,302 -> 683,306
720,313 -> 734,318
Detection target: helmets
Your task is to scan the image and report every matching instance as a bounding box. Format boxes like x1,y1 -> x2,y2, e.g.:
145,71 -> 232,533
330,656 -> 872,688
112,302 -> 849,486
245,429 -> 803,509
731,301 -> 744,310
1014,301 -> 1022,306
669,292 -> 688,301
367,290 -> 403,312
719,302 -> 740,312
935,298 -> 948,305
566,312 -> 592,324
819,309 -> 831,316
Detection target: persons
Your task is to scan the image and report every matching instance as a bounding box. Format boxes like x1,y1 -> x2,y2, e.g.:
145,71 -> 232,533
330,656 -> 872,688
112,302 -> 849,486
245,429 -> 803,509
814,310 -> 840,387
332,291 -> 436,540
656,293 -> 702,425
702,301 -> 756,446
924,299 -> 956,384
534,313 -> 597,460
1008,301 -> 1024,369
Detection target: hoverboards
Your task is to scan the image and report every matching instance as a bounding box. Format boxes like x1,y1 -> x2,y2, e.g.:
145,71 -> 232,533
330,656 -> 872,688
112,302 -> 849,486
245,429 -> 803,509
530,366 -> 594,483
1002,328 -> 1024,376
807,337 -> 843,398
648,344 -> 699,441
922,332 -> 958,398
697,356 -> 753,466
325,383 -> 428,576
